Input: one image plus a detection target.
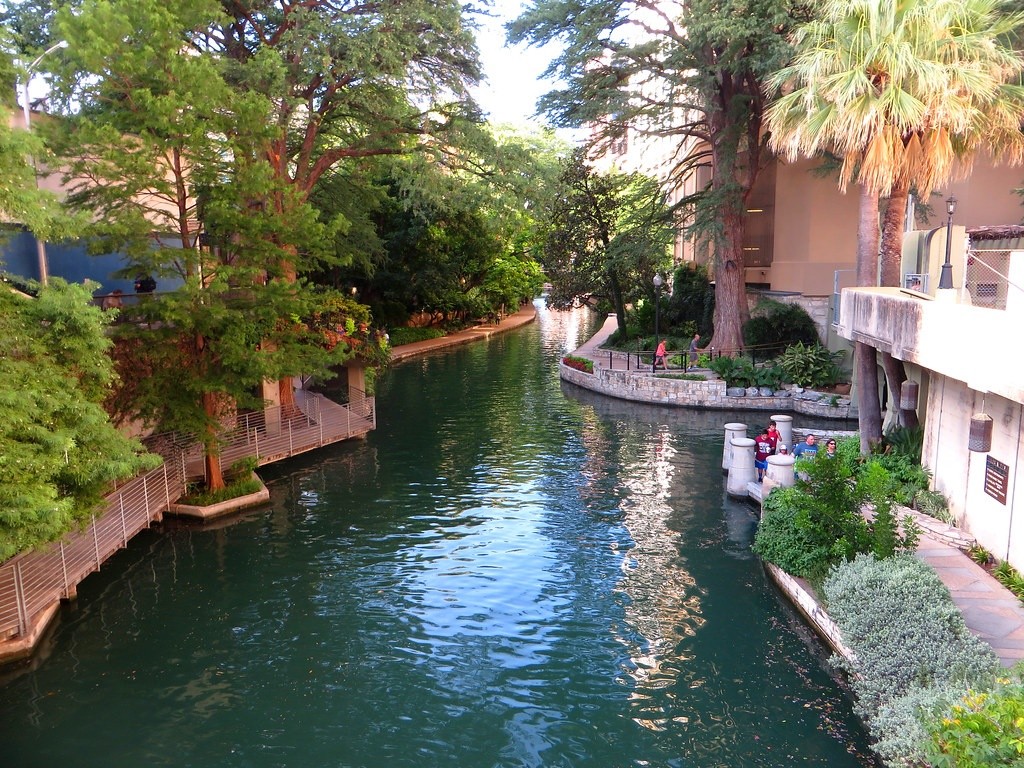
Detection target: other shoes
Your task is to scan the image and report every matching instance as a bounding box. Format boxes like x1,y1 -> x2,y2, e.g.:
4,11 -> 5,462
759,478 -> 763,482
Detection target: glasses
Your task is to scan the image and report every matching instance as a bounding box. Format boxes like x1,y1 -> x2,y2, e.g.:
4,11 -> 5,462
829,442 -> 836,445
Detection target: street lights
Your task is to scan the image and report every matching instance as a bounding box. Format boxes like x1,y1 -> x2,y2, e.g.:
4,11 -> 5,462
652,273 -> 664,363
935,191 -> 956,290
20,39 -> 73,296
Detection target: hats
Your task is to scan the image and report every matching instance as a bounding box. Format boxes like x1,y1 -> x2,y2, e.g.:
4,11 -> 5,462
779,444 -> 787,450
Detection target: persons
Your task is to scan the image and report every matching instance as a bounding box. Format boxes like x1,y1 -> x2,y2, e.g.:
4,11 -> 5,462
825,438 -> 837,460
102,289 -> 123,311
133,271 -> 156,295
488,310 -> 494,327
495,311 -> 501,327
778,445 -> 789,456
767,421 -> 782,455
687,333 -> 702,371
790,434 -> 818,481
520,296 -> 529,306
650,338 -> 669,371
755,429 -> 776,483
791,443 -> 799,480
